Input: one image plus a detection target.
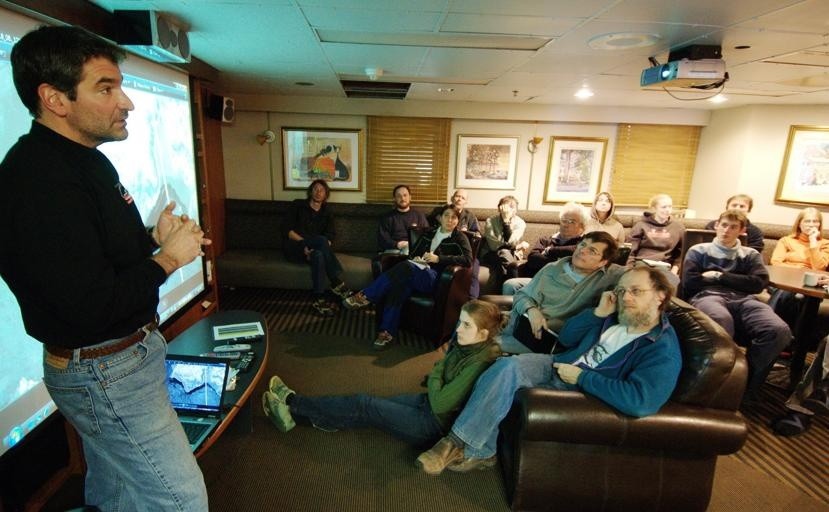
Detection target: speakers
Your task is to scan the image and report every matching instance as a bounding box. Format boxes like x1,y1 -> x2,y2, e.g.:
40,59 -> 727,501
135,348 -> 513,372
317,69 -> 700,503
114,10 -> 191,63
207,95 -> 235,121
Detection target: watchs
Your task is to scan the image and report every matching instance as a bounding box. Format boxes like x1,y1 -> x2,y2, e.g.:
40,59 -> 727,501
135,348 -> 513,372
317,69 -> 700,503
713,271 -> 718,279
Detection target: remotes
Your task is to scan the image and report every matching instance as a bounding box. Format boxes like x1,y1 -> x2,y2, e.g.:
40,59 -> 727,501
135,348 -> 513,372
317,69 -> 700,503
199,352 -> 240,360
236,351 -> 254,369
213,344 -> 251,353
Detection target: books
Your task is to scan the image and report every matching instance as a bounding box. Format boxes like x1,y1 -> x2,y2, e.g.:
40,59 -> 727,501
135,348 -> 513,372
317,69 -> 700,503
515,313 -> 560,355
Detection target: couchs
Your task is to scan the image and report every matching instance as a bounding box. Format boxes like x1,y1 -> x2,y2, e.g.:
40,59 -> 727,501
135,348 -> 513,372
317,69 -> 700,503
216,201 -> 829,329
483,275 -> 749,511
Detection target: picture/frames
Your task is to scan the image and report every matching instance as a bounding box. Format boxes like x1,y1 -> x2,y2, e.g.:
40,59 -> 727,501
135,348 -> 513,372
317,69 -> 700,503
455,134 -> 520,190
541,135 -> 609,206
772,122 -> 829,207
280,125 -> 364,192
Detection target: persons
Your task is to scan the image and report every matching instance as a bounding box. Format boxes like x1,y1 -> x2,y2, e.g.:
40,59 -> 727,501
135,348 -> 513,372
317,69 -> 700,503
683,209 -> 791,408
425,231 -> 632,380
0,26 -> 212,512
415,265 -> 682,476
373,185 -> 430,279
427,189 -> 482,257
703,195 -> 765,254
477,196 -> 529,269
589,193 -> 631,266
627,195 -> 684,297
768,207 -> 829,342
262,300 -> 513,444
342,204 -> 474,348
283,180 -> 356,315
518,201 -> 592,278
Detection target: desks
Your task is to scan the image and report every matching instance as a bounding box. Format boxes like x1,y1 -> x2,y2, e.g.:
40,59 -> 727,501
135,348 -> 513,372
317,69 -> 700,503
760,263 -> 829,392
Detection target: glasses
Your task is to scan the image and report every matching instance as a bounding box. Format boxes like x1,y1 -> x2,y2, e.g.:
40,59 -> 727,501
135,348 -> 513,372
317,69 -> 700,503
614,288 -> 660,296
559,219 -> 579,224
577,241 -> 604,257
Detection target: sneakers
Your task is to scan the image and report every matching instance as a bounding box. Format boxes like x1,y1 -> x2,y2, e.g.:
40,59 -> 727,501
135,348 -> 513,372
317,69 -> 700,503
773,411 -> 812,435
329,282 -> 353,299
263,392 -> 296,433
343,290 -> 372,311
415,437 -> 464,475
446,458 -> 497,471
269,376 -> 296,405
373,331 -> 397,351
312,296 -> 334,317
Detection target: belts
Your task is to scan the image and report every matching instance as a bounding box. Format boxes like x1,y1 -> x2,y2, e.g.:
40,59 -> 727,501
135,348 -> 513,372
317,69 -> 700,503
45,312 -> 160,359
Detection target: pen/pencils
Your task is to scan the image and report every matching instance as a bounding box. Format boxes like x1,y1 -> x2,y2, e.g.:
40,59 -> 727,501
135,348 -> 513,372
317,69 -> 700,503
537,318 -> 548,333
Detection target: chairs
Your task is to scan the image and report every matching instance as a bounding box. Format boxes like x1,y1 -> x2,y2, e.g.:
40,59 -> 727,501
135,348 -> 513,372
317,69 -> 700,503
371,229 -> 481,344
680,227 -> 748,294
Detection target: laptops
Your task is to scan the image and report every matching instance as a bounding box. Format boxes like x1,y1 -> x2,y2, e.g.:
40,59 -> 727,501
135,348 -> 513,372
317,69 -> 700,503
165,354 -> 229,453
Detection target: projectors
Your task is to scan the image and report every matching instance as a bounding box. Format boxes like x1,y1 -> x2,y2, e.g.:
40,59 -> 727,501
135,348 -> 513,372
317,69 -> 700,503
640,44 -> 729,89
